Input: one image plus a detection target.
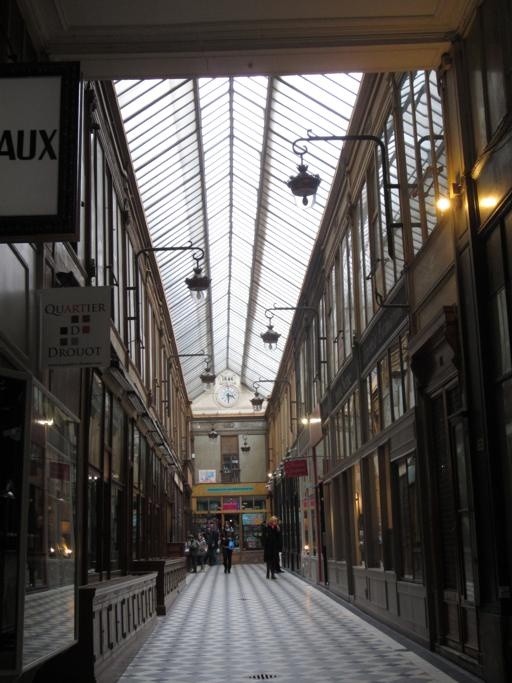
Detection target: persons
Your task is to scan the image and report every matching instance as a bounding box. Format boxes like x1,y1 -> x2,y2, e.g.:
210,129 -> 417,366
273,519 -> 284,573
260,516 -> 280,579
183,519 -> 236,573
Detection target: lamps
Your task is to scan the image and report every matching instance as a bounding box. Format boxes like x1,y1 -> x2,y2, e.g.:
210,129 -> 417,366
250,380 -> 297,433
166,349 -> 217,416
125,240 -> 211,343
288,129 -> 403,259
241,432 -> 274,470
261,303 -> 327,380
191,428 -> 218,459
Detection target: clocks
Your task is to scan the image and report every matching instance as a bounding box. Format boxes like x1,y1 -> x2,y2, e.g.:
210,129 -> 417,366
216,384 -> 239,408
